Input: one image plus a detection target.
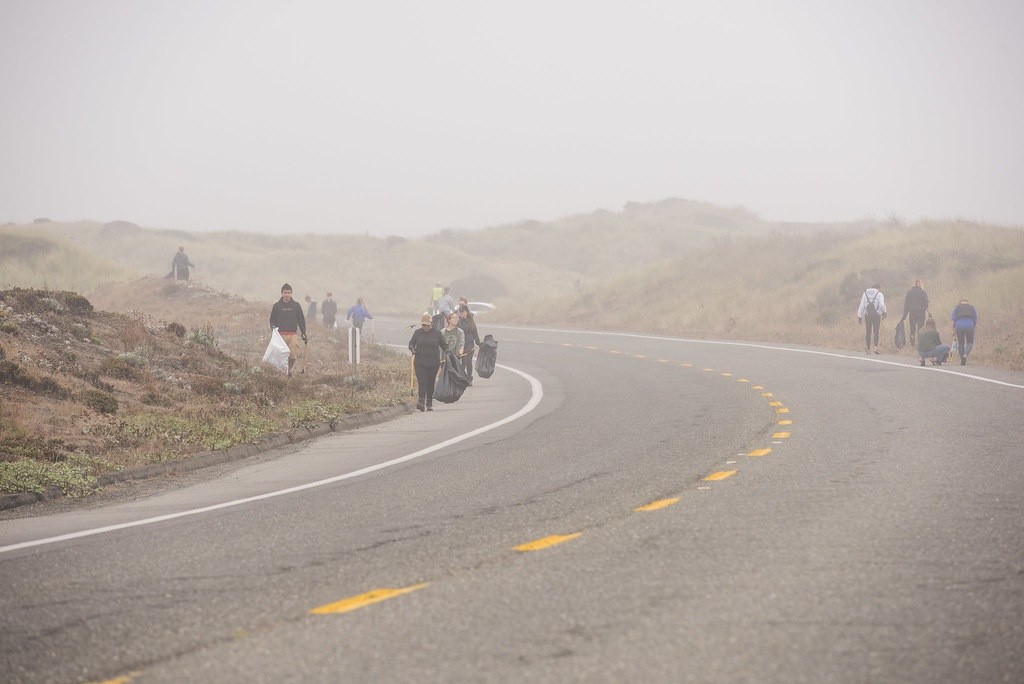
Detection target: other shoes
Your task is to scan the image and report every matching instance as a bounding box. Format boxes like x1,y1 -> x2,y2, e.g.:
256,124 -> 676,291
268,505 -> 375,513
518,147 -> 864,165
930,358 -> 941,366
961,356 -> 966,365
920,359 -> 925,366
417,404 -> 425,412
426,405 -> 433,411
874,348 -> 881,354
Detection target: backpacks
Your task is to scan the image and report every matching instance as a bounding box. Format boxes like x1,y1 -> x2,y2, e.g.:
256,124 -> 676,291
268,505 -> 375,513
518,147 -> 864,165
865,291 -> 881,320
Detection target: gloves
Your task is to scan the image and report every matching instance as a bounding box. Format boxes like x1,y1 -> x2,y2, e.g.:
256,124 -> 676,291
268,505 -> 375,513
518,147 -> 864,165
456,353 -> 463,358
272,325 -> 279,332
412,347 -> 416,355
445,348 -> 452,354
301,333 -> 307,340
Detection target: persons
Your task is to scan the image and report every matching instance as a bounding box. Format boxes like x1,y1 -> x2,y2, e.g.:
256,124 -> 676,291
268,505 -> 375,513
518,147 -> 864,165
172,247 -> 195,281
430,282 -> 444,315
409,312 -> 451,411
346,298 -> 373,335
437,286 -> 456,318
305,296 -> 317,321
916,318 -> 950,366
903,279 -> 929,346
321,292 -> 337,330
951,299 -> 977,366
858,285 -> 886,353
448,296 -> 481,386
440,313 -> 466,370
271,283 -> 306,378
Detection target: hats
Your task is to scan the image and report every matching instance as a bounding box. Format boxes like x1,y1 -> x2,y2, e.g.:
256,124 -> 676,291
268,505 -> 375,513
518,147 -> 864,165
420,313 -> 433,325
458,305 -> 468,311
